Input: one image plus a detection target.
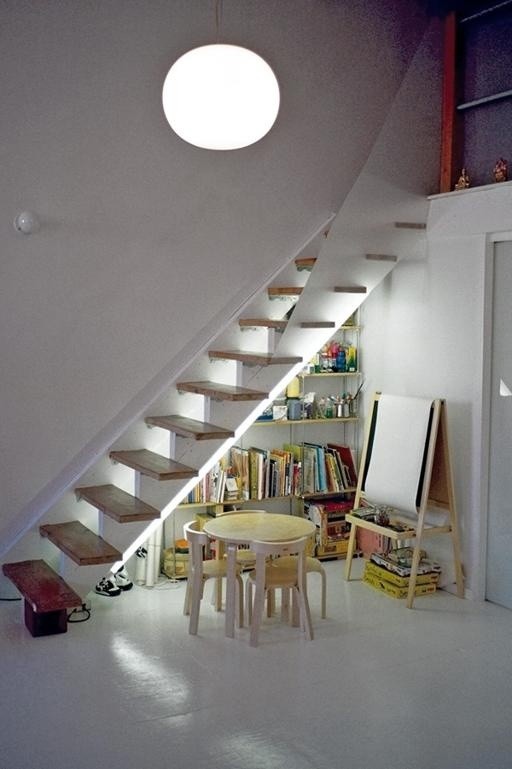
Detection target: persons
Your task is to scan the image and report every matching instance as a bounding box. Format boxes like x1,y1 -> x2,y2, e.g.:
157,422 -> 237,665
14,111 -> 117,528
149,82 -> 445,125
455,168 -> 471,189
493,158 -> 509,182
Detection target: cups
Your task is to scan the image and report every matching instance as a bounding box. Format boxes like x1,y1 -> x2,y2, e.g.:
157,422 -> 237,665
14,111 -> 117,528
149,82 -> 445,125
334,402 -> 350,418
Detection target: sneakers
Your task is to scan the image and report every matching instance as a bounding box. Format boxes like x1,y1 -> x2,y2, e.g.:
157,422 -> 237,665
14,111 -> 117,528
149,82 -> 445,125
94,576 -> 120,597
114,566 -> 133,590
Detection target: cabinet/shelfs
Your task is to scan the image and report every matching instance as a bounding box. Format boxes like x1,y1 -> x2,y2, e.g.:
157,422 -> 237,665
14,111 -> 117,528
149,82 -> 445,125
161,304 -> 364,578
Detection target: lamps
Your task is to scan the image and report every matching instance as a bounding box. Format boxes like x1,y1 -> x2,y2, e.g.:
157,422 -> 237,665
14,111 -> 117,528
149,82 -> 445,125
162,0 -> 281,156
13,209 -> 39,236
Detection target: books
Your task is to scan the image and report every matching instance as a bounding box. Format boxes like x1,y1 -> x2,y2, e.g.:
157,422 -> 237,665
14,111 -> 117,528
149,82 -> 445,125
176,441 -> 360,505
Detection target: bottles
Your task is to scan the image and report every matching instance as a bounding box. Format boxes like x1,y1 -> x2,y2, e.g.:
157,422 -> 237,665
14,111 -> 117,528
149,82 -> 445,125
286,377 -> 300,397
319,342 -> 358,373
287,396 -> 302,420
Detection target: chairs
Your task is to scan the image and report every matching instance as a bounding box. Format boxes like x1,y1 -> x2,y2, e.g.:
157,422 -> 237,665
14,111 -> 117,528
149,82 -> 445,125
181,507 -> 327,640
